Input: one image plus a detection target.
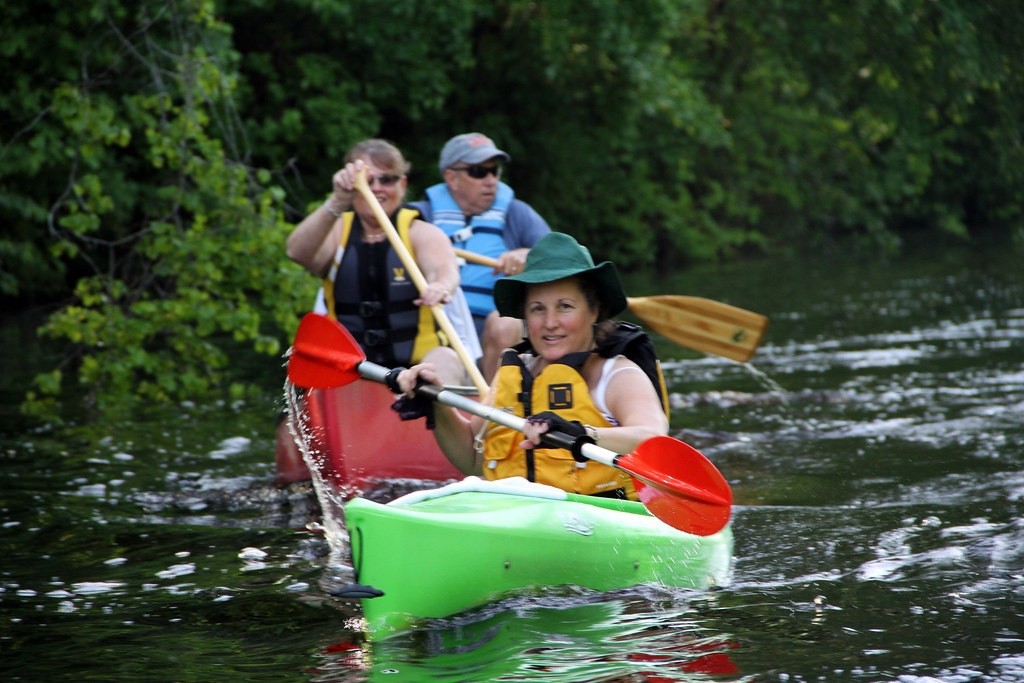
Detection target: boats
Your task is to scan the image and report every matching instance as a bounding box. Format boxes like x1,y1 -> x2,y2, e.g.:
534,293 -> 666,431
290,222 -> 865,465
278,379 -> 483,487
342,476 -> 733,645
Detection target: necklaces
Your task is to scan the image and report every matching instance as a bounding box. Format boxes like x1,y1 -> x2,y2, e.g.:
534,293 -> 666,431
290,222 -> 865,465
366,231 -> 384,238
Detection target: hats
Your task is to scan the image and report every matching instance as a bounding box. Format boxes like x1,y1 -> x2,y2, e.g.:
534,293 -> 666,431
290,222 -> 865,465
494,231 -> 627,325
439,133 -> 510,177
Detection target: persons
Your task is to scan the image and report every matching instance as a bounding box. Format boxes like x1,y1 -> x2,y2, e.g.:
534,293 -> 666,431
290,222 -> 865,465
285,138 -> 466,396
397,230 -> 670,502
405,131 -> 556,388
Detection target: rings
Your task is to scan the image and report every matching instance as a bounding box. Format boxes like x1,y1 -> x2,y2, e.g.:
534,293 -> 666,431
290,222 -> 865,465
511,267 -> 517,270
443,290 -> 449,295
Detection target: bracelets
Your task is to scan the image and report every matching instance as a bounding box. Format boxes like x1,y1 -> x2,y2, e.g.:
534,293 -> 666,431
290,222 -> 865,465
323,201 -> 341,218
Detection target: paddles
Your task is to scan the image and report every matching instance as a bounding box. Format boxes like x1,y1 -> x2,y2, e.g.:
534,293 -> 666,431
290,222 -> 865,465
352,164 -> 491,404
454,246 -> 769,364
286,311 -> 735,538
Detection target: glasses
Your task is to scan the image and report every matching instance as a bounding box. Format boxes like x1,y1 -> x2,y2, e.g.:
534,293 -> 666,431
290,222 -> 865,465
447,164 -> 503,179
367,175 -> 406,187
